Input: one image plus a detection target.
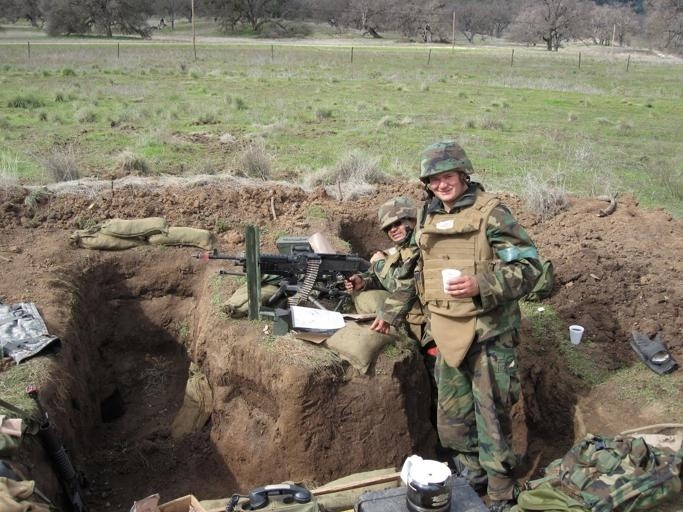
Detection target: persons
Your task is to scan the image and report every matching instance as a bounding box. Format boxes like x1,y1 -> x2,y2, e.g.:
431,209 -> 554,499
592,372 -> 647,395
370,140 -> 543,511
343,197 -> 437,397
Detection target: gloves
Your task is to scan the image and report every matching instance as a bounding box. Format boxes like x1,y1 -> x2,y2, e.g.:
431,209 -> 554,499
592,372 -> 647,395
629,340 -> 677,376
631,327 -> 670,364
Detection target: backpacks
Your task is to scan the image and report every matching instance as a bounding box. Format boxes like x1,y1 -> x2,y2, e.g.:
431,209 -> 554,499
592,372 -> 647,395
524,432 -> 682,512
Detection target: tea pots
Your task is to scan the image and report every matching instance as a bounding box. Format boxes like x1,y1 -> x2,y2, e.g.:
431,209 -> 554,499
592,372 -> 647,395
405,460 -> 451,512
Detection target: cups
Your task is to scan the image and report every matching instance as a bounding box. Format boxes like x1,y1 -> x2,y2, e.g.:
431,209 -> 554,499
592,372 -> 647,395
441,269 -> 461,295
568,325 -> 584,345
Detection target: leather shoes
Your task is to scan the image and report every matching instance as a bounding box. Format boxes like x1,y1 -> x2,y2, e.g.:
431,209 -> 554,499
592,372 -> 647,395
485,493 -> 507,512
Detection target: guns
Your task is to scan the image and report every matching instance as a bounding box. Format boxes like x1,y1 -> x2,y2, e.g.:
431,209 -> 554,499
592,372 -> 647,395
220,270 -> 349,313
25,383 -> 92,512
193,251 -> 372,306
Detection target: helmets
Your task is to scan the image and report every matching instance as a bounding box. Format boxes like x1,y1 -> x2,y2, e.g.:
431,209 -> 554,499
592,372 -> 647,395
420,141 -> 474,183
377,197 -> 418,230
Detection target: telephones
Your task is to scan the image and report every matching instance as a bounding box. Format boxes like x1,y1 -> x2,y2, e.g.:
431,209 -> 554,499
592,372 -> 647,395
250,484 -> 312,509
